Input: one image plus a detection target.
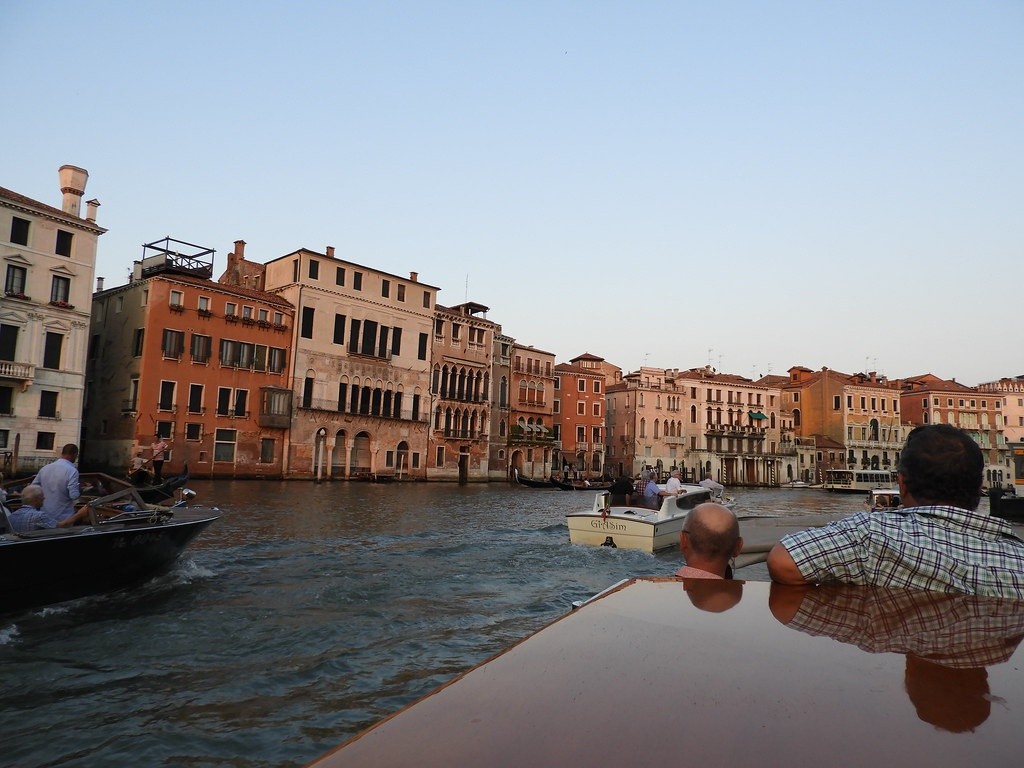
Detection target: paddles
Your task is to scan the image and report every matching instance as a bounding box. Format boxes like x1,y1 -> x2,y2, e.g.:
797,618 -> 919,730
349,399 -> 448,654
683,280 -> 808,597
133,448 -> 165,474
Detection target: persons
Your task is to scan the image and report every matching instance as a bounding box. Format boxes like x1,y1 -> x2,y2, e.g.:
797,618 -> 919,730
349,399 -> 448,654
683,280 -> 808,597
699,473 -> 723,503
0,473 -> 22,502
679,502 -> 745,584
640,459 -> 655,479
636,471 -> 649,508
564,462 -> 570,482
665,470 -> 687,500
644,472 -> 677,509
682,580 -> 744,613
150,433 -> 175,484
606,476 -> 635,507
584,478 -> 591,487
767,423 -> 1022,604
129,452 -> 151,470
572,460 -> 578,481
769,582 -> 1023,735
8,484 -> 89,532
31,443 -> 80,526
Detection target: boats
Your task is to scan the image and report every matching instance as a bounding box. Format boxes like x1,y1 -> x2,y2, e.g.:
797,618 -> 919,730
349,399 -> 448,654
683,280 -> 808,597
565,484 -> 738,555
517,474 -> 559,488
0,450 -> 223,615
549,474 -> 611,491
823,469 -> 906,513
